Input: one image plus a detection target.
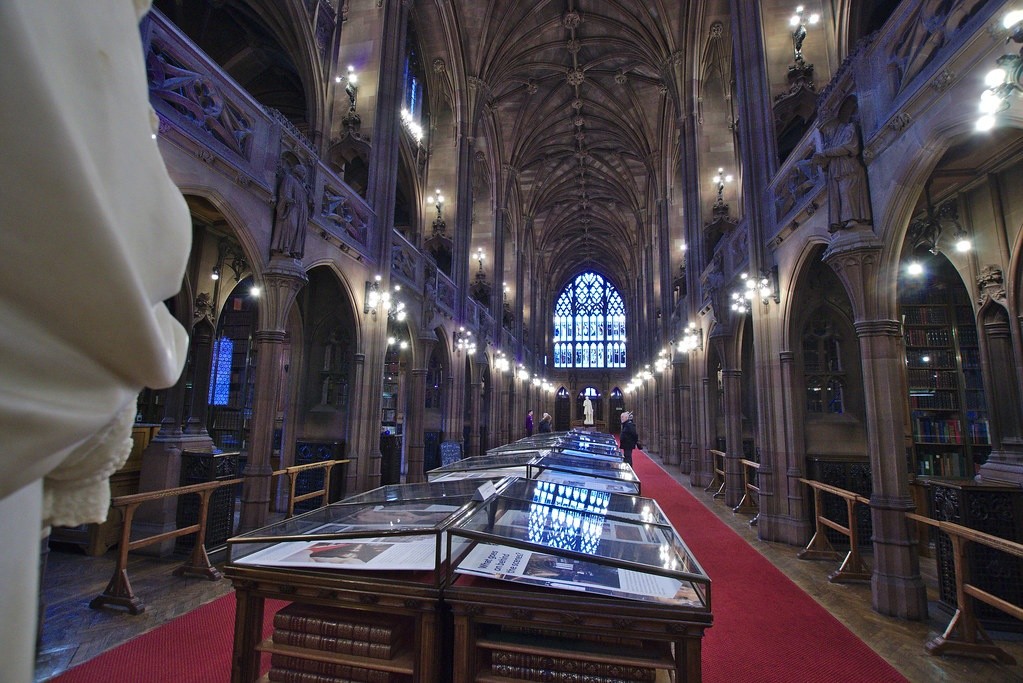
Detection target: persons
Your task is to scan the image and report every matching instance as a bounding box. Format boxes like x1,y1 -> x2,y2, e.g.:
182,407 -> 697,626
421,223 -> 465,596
620,413 -> 643,468
584,397 -> 594,424
422,277 -> 437,329
525,410 -> 533,437
812,108 -> 870,231
538,413 -> 553,433
270,166 -> 315,259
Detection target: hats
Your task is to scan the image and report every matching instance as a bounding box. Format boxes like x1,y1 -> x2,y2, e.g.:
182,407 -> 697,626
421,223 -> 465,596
637,442 -> 643,450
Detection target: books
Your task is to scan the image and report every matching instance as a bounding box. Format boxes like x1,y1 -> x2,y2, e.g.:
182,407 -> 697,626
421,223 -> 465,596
210,324 -> 251,451
897,284 -> 993,481
381,352 -> 398,436
804,340 -> 842,412
268,603 -> 657,683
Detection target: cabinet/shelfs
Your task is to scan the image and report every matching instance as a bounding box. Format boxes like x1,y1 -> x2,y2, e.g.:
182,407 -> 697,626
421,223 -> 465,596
222,566 -> 441,683
321,329 -> 401,425
900,265 -> 986,557
207,293 -> 282,458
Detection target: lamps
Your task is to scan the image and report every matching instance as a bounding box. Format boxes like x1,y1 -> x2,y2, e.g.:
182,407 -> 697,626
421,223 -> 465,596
712,167 -> 732,193
453,325 -> 475,356
427,189 -> 443,216
790,4 -> 820,51
741,265 -> 781,305
364,274 -> 407,321
212,233 -> 258,299
473,247 -> 486,268
676,321 -> 703,354
907,178 -> 971,273
732,288 -> 752,316
972,8 -> 1023,132
653,348 -> 672,373
335,64 -> 359,103
